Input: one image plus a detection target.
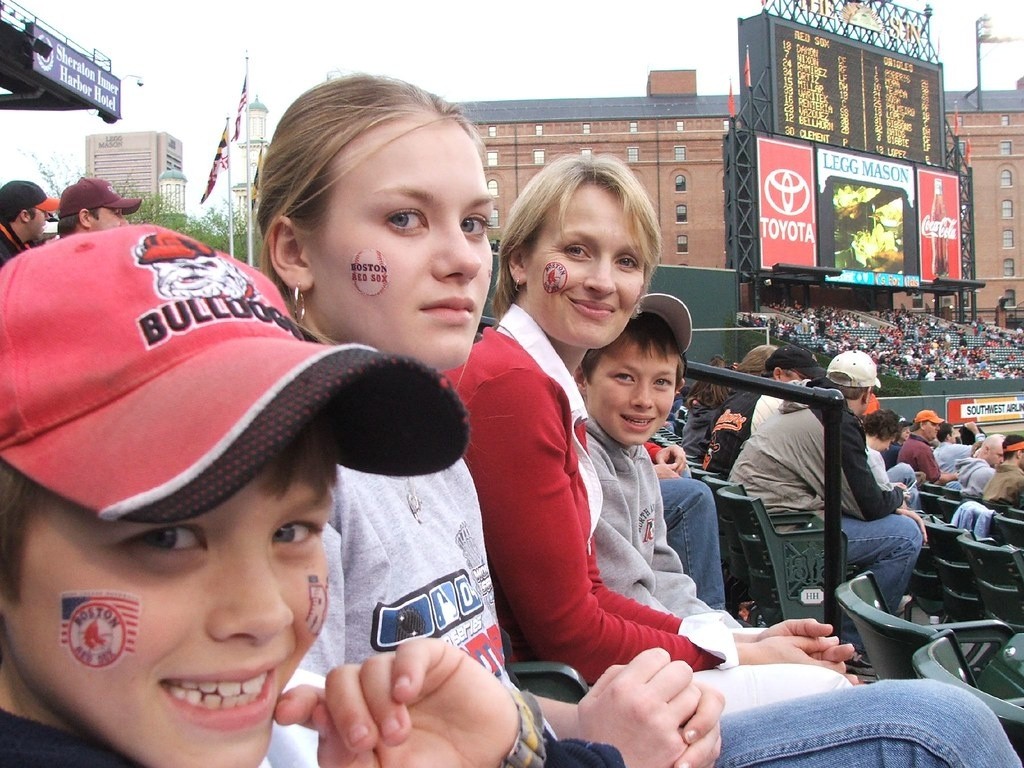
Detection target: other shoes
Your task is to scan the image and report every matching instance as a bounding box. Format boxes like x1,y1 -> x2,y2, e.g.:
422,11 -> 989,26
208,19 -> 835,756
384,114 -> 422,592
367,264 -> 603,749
896,594 -> 913,617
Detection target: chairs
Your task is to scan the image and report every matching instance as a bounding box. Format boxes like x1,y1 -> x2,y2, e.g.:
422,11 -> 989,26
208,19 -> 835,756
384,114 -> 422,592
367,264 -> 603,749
651,421 -> 1024,745
774,324 -> 1023,380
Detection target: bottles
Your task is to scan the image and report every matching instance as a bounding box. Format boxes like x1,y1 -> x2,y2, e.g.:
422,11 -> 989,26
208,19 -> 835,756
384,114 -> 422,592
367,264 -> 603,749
927,177 -> 950,276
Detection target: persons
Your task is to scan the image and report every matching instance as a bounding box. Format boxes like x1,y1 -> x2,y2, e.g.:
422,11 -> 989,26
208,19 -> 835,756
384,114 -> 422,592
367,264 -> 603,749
728,350 -> 924,616
0,179 -> 60,266
879,415 -> 912,471
1,223 -> 627,768
57,176 -> 142,239
701,344 -> 826,482
932,421 -> 986,474
863,409 -> 921,517
970,440 -> 994,468
981,434 -> 1024,511
666,385 -> 689,437
253,76 -> 1023,768
441,152 -> 856,718
955,435 -> 1004,497
743,298 -> 1024,381
897,409 -> 964,491
572,293 -> 747,630
682,354 -> 736,482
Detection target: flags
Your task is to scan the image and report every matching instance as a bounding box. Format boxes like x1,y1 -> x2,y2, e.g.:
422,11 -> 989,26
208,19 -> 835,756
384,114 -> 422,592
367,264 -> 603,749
199,122 -> 230,205
252,150 -> 261,210
231,76 -> 248,144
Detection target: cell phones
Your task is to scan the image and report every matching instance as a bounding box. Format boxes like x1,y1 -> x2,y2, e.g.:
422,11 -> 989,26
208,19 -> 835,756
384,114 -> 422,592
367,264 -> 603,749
903,476 -> 917,486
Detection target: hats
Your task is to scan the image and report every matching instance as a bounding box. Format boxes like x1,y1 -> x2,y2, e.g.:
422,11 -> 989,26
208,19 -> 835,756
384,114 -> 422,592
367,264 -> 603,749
640,292 -> 692,356
826,350 -> 882,389
898,415 -> 913,426
765,344 -> 828,380
0,180 -> 60,218
0,223 -> 469,523
861,392 -> 879,416
59,176 -> 142,219
916,409 -> 944,424
